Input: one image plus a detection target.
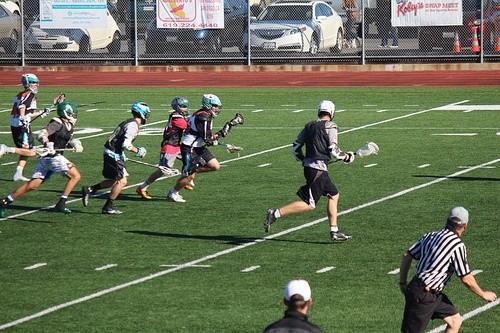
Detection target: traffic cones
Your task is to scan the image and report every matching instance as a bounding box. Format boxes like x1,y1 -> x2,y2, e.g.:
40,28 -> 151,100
453,30 -> 461,53
471,30 -> 480,52
496,37 -> 500,52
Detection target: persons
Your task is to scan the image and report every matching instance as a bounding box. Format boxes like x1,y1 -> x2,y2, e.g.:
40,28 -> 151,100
340,0 -> 362,48
0,102 -> 83,217
377,0 -> 398,48
136,97 -> 194,199
0,73 -> 49,182
264,100 -> 355,241
167,94 -> 227,202
398,207 -> 497,333
262,279 -> 324,333
81,101 -> 151,214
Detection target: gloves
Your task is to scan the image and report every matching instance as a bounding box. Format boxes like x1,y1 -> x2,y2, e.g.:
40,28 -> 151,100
344,151 -> 355,165
70,139 -> 84,153
20,116 -> 28,129
134,146 -> 147,158
46,142 -> 56,155
218,122 -> 231,138
39,107 -> 50,118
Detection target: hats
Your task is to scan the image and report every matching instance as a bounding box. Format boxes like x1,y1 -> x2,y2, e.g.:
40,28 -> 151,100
284,280 -> 312,302
449,206 -> 469,225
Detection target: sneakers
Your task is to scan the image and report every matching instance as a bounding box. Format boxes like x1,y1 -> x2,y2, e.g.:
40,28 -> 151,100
0,143 -> 6,159
167,191 -> 186,202
185,185 -> 194,191
53,205 -> 71,214
136,186 -> 151,199
264,209 -> 277,233
13,174 -> 31,182
189,179 -> 196,187
82,185 -> 93,207
331,231 -> 351,240
102,206 -> 123,214
0,200 -> 7,218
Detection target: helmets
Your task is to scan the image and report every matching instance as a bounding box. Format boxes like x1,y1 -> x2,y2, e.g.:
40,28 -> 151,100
58,102 -> 78,125
132,101 -> 151,125
202,93 -> 222,118
171,97 -> 191,119
21,73 -> 40,95
317,100 -> 335,120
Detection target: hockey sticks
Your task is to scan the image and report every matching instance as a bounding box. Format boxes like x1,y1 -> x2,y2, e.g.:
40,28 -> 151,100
328,142 -> 380,165
221,112 -> 245,137
29,95 -> 67,122
126,157 -> 181,177
218,143 -> 244,154
31,146 -> 75,156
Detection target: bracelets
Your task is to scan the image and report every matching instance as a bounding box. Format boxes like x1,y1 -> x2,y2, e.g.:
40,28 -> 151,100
399,281 -> 408,285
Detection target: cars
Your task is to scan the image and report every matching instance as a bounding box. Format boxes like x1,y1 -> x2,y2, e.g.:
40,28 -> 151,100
0,0 -> 343,55
419,0 -> 500,52
